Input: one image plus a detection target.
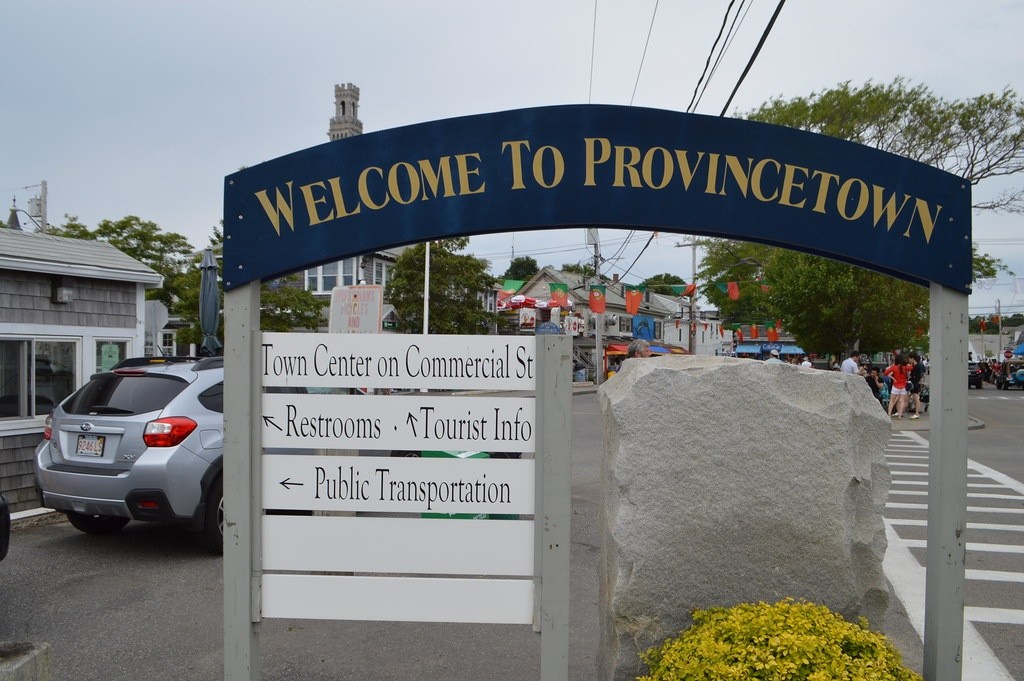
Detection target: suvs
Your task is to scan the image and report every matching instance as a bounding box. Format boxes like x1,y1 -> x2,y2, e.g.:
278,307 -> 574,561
967,358 -> 1024,390
31,353 -> 423,556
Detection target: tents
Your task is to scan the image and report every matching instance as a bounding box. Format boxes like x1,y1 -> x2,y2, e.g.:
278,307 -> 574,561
534,298 -> 573,309
499,295 -> 548,310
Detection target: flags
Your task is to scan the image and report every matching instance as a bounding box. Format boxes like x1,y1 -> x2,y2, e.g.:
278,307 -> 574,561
716,282 -> 740,300
498,280 -> 524,302
765,321 -> 779,343
670,286 -> 695,297
589,285 -> 606,314
750,323 -> 759,340
692,320 -> 696,332
704,323 -> 709,331
720,325 -> 724,338
548,284 -> 568,307
732,323 -> 743,343
625,286 -> 645,315
676,319 -> 679,329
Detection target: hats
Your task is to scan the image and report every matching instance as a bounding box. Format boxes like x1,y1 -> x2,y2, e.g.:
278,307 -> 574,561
771,350 -> 778,355
802,357 -> 809,361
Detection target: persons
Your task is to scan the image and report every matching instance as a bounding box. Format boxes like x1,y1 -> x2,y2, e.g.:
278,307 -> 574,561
789,351 -> 1024,387
908,352 -> 922,420
884,355 -> 913,422
839,351 -> 863,376
864,367 -> 884,406
766,350 -> 783,363
614,339 -> 651,373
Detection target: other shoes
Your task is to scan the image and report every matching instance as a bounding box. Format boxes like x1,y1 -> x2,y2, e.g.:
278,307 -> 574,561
889,412 -> 919,420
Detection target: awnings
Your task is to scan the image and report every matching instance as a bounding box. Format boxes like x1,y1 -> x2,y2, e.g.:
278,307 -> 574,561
735,345 -> 761,353
606,344 -> 689,356
778,345 -> 805,355
